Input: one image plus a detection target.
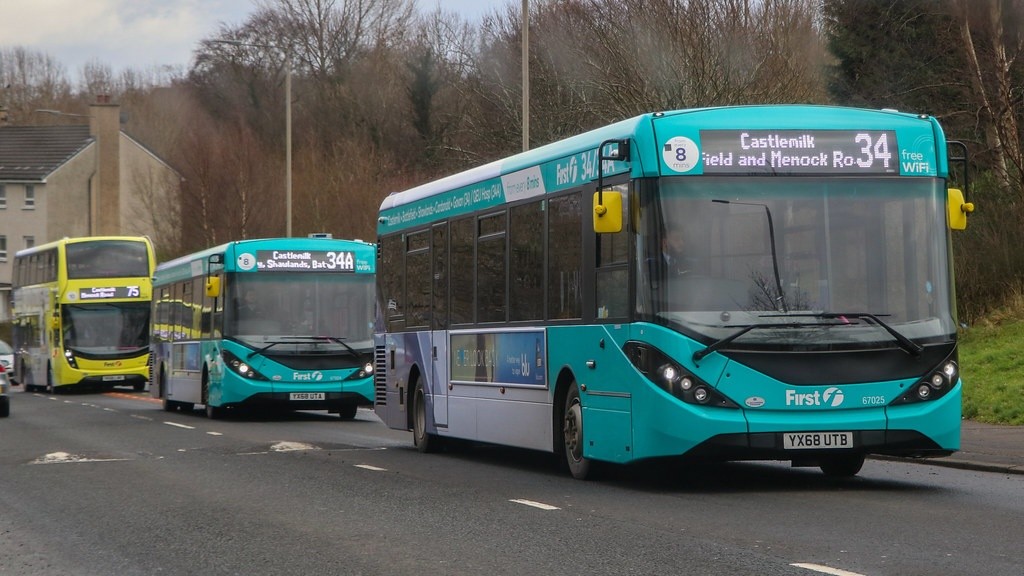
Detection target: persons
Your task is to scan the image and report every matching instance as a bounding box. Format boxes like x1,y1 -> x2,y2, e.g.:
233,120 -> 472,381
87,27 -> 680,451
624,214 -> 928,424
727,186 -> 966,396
234,288 -> 266,318
619,223 -> 702,312
297,299 -> 331,337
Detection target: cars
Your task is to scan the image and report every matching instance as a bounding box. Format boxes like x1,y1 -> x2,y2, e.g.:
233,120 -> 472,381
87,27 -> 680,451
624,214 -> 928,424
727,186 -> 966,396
0,339 -> 15,418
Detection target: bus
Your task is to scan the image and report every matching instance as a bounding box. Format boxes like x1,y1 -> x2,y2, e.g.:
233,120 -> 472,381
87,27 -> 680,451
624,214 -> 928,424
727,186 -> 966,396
148,233 -> 377,420
149,293 -> 223,343
11,238 -> 158,395
373,104 -> 976,478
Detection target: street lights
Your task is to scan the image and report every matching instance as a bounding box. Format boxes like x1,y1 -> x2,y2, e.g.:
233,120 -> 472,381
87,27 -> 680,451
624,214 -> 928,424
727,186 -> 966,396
210,38 -> 293,238
35,108 -> 102,238
